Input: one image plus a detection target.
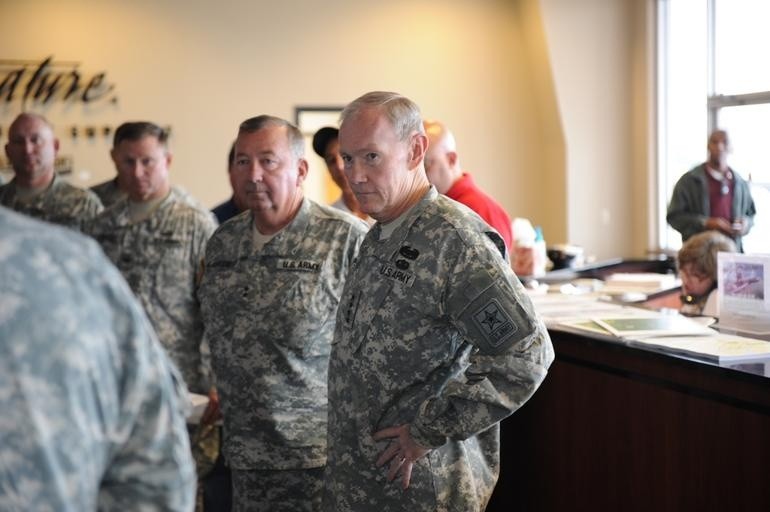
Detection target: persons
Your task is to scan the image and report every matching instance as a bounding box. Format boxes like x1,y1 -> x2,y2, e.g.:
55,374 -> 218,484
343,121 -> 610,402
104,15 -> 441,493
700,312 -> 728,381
665,129 -> 756,253
319,91 -> 556,511
209,139 -> 249,224
196,114 -> 371,510
418,117 -> 514,260
82,119 -> 222,511
89,122 -> 132,210
678,229 -> 737,317
312,123 -> 377,231
0,203 -> 199,511
0,109 -> 105,229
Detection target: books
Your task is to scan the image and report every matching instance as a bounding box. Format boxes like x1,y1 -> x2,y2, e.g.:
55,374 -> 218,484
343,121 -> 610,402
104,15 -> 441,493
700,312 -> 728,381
528,269 -> 769,364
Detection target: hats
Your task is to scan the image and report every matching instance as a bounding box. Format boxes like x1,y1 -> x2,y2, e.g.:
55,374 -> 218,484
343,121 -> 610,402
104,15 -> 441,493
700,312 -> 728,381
313,126 -> 338,156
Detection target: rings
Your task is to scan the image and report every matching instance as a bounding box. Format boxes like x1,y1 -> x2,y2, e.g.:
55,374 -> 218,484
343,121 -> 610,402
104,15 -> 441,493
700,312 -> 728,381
396,454 -> 406,464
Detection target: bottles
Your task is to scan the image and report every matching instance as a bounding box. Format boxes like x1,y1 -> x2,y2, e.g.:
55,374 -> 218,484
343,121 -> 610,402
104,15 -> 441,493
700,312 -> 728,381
532,226 -> 547,280
